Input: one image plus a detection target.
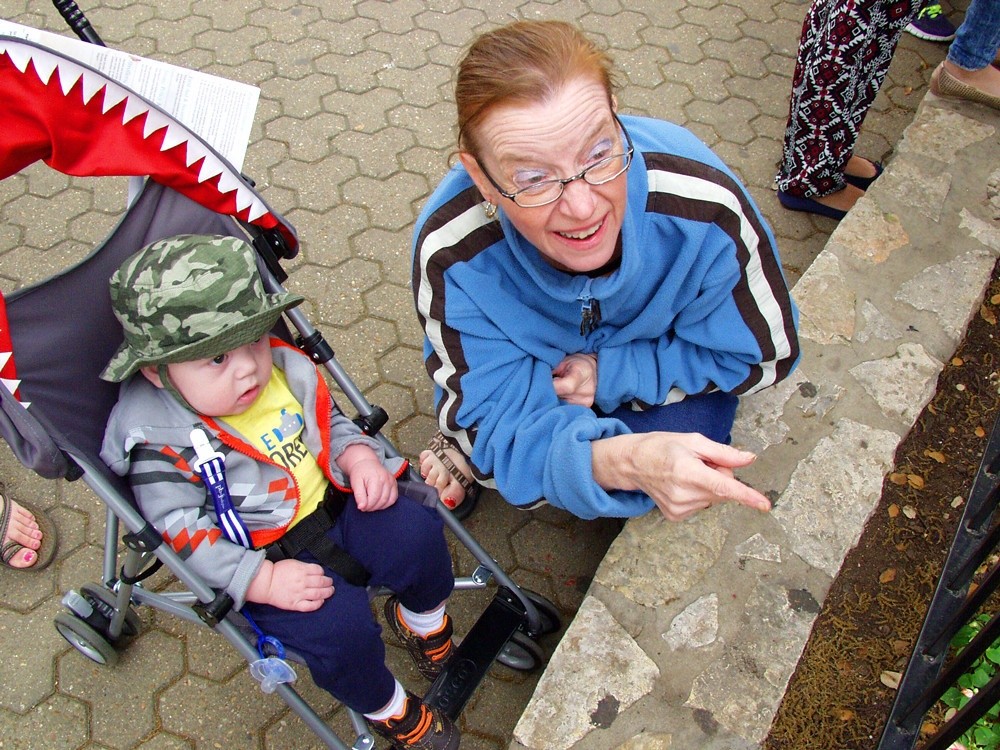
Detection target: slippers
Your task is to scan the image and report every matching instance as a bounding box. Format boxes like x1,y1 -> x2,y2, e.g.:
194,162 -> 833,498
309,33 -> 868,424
0,491 -> 58,571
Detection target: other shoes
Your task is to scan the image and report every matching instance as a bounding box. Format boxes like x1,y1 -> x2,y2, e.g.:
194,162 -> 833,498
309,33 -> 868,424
777,184 -> 868,223
928,61 -> 1000,112
844,153 -> 884,191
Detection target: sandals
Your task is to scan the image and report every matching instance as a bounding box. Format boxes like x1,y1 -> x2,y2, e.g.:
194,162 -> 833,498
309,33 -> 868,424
417,429 -> 480,521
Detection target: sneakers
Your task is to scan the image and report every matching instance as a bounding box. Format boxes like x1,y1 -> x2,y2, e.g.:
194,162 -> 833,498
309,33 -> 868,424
364,690 -> 462,749
382,598 -> 456,680
903,0 -> 957,42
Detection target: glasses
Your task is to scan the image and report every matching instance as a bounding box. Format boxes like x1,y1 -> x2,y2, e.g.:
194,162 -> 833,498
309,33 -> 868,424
469,111 -> 633,208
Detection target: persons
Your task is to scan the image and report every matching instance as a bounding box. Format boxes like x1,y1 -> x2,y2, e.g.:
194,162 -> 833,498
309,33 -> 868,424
930,0 -> 999,111
94,231 -> 465,750
415,18 -> 805,525
903,0 -> 959,45
1,478 -> 63,573
776,1 -> 926,220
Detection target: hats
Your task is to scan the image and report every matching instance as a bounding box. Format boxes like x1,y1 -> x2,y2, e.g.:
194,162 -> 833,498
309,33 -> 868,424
98,233 -> 305,415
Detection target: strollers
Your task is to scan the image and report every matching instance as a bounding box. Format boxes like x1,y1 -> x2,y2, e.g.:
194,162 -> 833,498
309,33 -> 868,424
2,0 -> 562,750
